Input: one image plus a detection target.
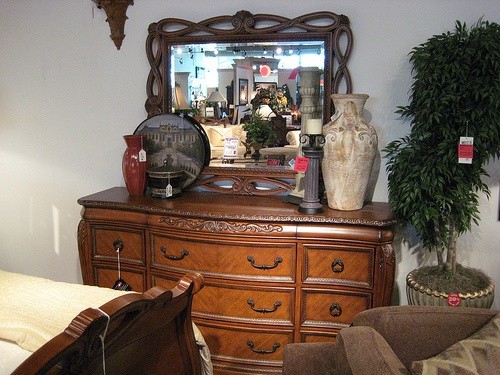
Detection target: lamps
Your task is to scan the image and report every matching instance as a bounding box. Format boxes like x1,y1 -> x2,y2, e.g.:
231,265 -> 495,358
296,45 -> 302,55
179,58 -> 184,64
174,82 -> 193,112
232,46 -> 240,54
263,48 -> 267,55
242,49 -> 247,57
317,46 -> 322,54
205,87 -> 226,120
190,54 -> 194,59
288,45 -> 294,55
188,47 -> 193,54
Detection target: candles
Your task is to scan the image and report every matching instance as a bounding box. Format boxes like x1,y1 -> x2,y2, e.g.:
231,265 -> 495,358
305,117 -> 324,134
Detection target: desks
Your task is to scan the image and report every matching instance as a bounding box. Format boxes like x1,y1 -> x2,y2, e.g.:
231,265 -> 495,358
199,121 -> 248,146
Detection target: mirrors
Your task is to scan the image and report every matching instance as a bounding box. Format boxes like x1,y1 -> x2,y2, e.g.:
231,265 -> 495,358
142,9 -> 355,196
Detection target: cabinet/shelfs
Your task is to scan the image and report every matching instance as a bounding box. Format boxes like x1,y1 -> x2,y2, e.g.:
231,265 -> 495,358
76,185 -> 396,375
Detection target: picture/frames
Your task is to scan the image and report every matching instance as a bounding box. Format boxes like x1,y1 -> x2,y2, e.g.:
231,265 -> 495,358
238,78 -> 249,107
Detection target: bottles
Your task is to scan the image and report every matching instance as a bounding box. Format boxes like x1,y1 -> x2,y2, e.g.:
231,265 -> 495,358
319,94 -> 378,212
122,135 -> 148,196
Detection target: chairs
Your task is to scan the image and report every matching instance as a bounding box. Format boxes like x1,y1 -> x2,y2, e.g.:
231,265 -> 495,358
6,272 -> 206,375
258,128 -> 301,160
0,271 -> 213,375
281,304 -> 500,375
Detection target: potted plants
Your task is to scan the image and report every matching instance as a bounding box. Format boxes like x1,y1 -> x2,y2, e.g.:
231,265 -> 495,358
242,106 -> 278,159
379,13 -> 500,308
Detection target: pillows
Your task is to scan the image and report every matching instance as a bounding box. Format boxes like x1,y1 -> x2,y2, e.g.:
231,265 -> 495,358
408,309 -> 500,375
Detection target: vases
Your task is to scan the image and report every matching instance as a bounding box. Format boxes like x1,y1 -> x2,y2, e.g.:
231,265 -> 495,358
120,134 -> 148,197
320,93 -> 377,213
